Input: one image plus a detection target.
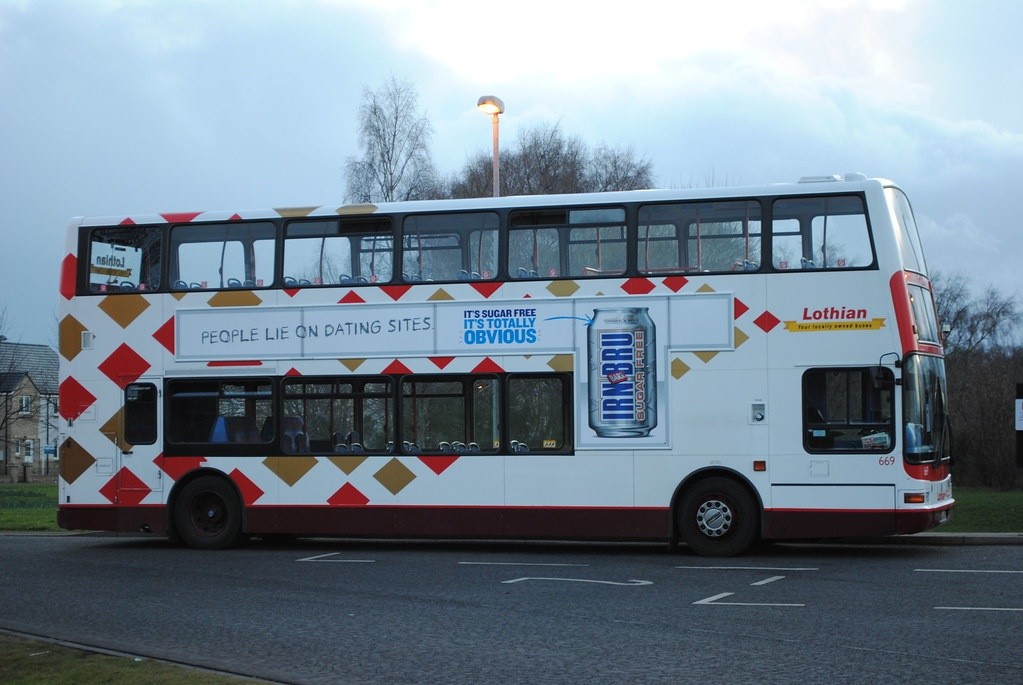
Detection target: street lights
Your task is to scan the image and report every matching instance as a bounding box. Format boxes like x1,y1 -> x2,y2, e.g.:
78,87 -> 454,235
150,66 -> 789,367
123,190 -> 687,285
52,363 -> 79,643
476,94 -> 506,196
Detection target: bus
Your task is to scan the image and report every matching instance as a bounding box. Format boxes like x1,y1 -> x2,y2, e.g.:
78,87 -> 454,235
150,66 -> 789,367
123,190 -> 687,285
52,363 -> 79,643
51,170 -> 957,562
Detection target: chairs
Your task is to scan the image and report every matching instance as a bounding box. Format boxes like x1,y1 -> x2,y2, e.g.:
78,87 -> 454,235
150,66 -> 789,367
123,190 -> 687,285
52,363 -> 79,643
223,415 -> 530,453
119,258 -> 847,291
